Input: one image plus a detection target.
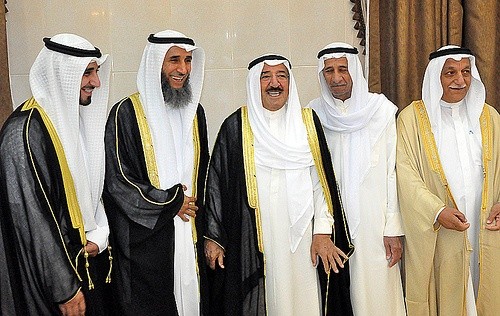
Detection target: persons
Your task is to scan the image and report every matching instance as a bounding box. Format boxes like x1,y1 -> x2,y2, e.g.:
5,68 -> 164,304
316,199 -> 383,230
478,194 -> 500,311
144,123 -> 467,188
397,46 -> 500,316
103,29 -> 209,316
0,34 -> 118,316
203,53 -> 356,316
307,42 -> 406,316
